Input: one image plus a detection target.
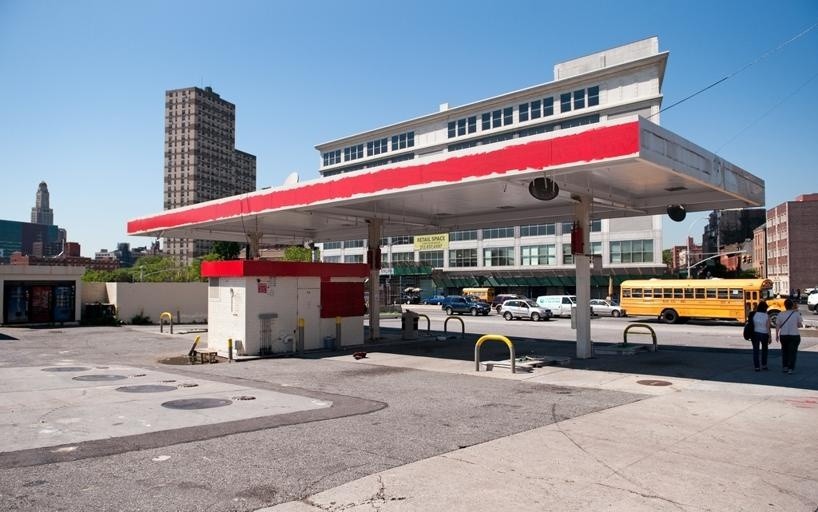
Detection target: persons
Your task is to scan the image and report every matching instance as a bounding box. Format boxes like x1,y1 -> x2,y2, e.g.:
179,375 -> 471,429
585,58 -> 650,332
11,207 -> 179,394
790,289 -> 795,299
797,287 -> 801,299
776,293 -> 781,298
748,301 -> 772,372
775,299 -> 804,374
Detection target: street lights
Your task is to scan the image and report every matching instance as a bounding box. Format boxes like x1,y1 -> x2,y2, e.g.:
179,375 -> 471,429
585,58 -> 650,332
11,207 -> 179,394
379,239 -> 409,280
687,215 -> 711,279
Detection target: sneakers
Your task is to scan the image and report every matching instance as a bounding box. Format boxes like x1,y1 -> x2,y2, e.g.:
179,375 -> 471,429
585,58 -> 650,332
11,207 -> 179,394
784,367 -> 793,373
755,366 -> 768,371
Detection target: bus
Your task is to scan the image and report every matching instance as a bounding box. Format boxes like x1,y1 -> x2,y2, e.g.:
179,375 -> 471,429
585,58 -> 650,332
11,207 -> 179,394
458,288 -> 496,305
619,278 -> 798,328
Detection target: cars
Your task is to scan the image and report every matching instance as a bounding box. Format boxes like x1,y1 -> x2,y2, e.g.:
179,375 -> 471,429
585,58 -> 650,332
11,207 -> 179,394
422,295 -> 445,305
807,290 -> 818,314
589,298 -> 626,318
392,294 -> 420,305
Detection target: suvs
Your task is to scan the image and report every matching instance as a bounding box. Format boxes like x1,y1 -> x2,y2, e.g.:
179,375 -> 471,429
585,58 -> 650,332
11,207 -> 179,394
441,295 -> 491,316
492,294 -> 528,314
500,299 -> 553,321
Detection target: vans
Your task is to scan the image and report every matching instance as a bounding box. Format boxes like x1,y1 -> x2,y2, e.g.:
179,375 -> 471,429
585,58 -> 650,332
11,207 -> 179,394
535,295 -> 576,317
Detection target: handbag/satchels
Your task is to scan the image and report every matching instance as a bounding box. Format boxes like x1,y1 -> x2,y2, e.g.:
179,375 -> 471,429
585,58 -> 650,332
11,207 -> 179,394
744,322 -> 754,340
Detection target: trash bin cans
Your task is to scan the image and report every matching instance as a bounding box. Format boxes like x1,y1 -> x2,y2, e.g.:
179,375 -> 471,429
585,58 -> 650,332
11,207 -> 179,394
401,309 -> 419,339
324,336 -> 336,350
